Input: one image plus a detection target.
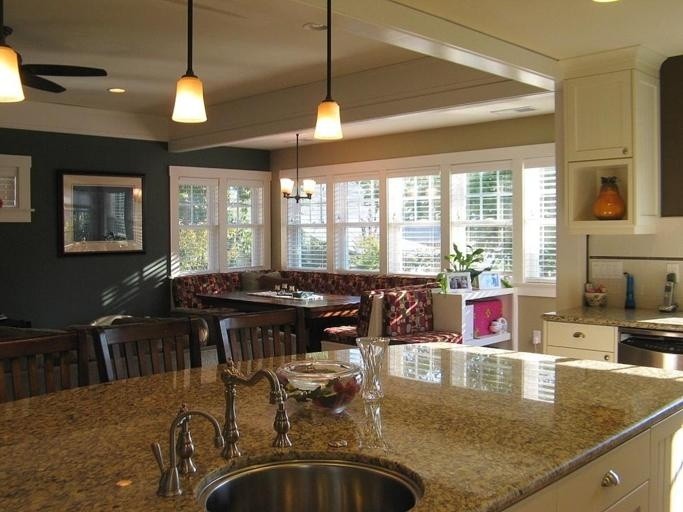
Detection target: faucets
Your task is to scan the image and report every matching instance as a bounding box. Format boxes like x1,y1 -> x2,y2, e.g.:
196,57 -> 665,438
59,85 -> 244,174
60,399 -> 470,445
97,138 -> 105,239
146,409 -> 230,498
216,353 -> 284,460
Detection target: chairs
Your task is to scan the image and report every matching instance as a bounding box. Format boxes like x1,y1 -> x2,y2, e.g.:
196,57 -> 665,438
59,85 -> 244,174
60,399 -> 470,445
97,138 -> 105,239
1,329 -> 89,402
87,320 -> 201,381
212,307 -> 306,363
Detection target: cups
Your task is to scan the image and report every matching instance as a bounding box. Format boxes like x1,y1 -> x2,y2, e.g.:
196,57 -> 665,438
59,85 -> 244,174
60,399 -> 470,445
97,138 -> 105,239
362,400 -> 383,446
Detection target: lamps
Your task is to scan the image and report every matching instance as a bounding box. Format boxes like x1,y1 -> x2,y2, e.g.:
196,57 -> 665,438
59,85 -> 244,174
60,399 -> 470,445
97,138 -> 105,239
279,134 -> 315,204
1,1 -> 24,103
314,1 -> 343,142
171,2 -> 207,124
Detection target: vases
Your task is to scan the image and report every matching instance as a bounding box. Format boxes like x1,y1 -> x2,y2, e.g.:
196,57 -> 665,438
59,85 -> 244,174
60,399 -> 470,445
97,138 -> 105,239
589,174 -> 628,219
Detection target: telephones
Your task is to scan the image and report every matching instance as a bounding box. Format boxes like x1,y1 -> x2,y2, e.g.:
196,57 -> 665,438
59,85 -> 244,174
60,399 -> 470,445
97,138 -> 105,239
659,281 -> 679,313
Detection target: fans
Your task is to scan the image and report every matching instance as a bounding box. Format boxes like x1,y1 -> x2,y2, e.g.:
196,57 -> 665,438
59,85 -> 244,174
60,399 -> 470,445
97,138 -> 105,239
2,23 -> 108,94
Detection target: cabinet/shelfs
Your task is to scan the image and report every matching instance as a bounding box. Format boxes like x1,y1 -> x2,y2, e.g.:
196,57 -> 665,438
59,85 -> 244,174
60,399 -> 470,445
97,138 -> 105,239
499,388 -> 682,512
562,68 -> 660,235
431,288 -> 519,353
538,317 -> 622,363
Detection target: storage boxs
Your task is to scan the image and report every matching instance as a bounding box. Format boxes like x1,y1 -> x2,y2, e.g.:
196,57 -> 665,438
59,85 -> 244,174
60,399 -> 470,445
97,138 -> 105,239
468,298 -> 503,339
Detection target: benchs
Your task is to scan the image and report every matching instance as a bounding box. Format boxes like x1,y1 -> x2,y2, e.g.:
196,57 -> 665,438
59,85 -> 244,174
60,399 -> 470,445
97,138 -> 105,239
168,266 -> 462,351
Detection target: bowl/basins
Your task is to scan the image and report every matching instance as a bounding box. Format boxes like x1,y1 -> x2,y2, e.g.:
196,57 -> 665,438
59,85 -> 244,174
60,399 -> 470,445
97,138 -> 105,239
584,290 -> 611,308
287,409 -> 360,443
274,360 -> 361,407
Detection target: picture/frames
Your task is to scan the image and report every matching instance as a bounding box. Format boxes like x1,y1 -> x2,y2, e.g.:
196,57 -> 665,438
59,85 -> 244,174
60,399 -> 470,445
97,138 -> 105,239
444,272 -> 471,292
478,271 -> 499,289
53,170 -> 145,256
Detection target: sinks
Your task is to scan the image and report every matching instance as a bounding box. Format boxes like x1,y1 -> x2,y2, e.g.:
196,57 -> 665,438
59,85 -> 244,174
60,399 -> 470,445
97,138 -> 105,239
192,449 -> 427,512
